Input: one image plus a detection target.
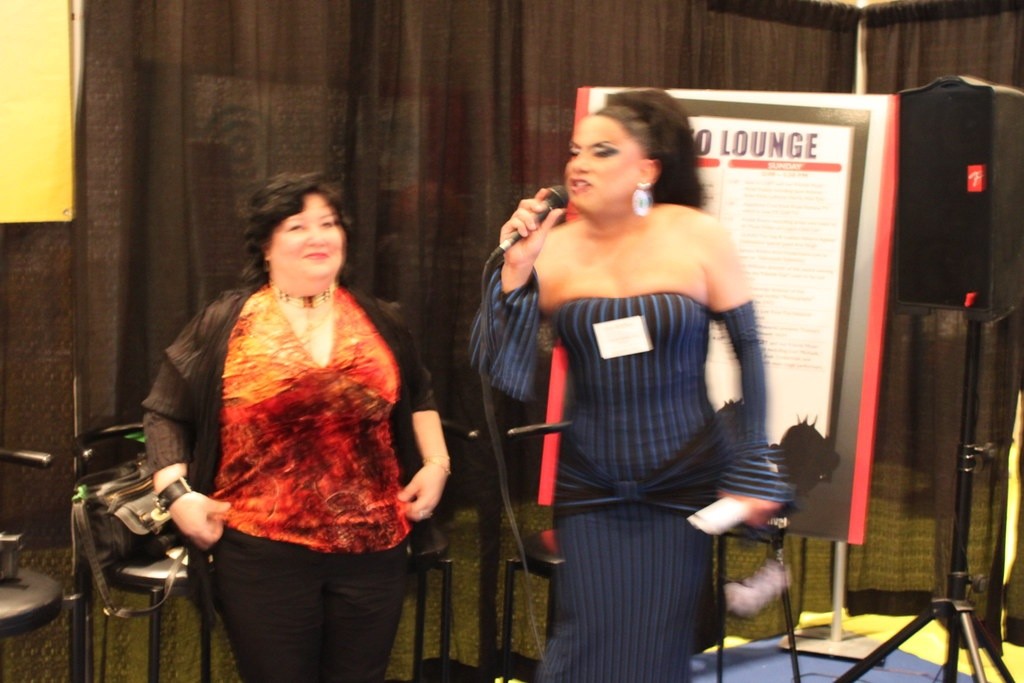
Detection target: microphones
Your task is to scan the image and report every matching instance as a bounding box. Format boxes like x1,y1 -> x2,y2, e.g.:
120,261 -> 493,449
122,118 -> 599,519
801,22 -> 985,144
488,186 -> 570,261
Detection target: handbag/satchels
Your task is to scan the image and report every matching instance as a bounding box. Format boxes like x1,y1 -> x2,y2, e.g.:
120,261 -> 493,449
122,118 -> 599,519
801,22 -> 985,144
72,451 -> 189,620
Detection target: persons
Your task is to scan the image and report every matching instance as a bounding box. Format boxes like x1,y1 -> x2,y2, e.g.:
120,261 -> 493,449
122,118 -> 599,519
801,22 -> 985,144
468,89 -> 790,683
141,171 -> 451,683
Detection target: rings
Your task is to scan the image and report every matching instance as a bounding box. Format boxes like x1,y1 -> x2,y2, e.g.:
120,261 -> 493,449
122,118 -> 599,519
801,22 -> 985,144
420,510 -> 431,519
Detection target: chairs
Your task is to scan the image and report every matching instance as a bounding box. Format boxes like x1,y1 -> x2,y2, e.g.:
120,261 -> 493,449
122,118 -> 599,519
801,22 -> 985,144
410,526 -> 453,683
0,421 -> 215,683
502,528 -> 566,683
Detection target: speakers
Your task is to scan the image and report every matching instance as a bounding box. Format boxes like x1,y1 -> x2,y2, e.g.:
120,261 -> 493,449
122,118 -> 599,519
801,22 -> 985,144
890,75 -> 1024,315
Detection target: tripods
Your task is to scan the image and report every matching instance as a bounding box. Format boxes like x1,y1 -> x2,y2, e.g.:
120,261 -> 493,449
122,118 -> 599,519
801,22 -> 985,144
833,311 -> 1016,683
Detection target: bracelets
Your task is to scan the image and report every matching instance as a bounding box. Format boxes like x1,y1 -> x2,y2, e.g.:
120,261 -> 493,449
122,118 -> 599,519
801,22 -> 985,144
153,476 -> 191,513
422,454 -> 450,474
